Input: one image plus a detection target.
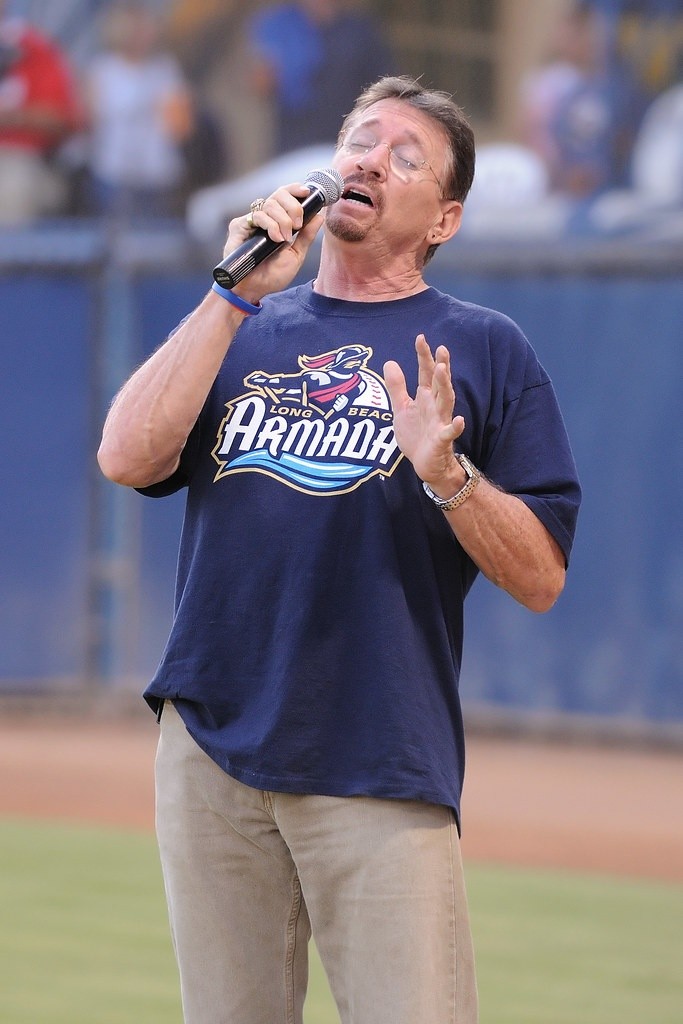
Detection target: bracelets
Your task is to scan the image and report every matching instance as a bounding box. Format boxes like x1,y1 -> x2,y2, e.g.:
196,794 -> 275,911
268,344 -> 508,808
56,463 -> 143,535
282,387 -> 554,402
213,281 -> 263,316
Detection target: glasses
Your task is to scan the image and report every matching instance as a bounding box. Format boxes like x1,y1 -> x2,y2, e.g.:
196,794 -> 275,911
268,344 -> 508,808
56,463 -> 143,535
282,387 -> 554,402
339,129 -> 448,200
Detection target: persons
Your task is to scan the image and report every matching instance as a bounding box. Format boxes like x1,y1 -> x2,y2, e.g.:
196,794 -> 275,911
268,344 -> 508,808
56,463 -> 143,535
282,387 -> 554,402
97,68 -> 584,1022
1,0 -> 683,243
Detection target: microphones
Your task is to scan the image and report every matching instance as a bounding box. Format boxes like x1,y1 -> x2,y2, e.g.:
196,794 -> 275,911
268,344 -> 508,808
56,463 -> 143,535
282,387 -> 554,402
212,167 -> 345,290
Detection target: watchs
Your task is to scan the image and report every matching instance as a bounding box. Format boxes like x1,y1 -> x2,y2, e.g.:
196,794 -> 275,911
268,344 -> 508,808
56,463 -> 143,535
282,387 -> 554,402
424,451 -> 485,510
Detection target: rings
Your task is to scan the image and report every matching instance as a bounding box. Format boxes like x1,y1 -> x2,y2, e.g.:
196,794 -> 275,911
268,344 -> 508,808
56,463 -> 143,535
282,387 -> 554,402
247,212 -> 255,229
250,199 -> 265,216
